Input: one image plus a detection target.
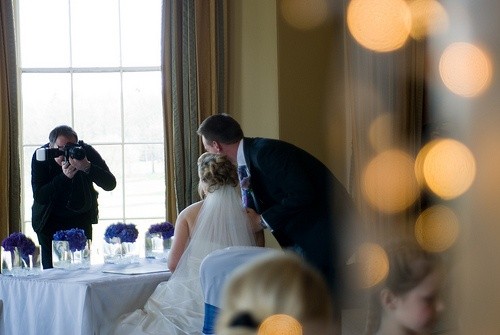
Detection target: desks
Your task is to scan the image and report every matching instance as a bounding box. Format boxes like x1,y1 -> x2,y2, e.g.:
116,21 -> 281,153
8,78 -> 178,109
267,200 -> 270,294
0,257 -> 172,335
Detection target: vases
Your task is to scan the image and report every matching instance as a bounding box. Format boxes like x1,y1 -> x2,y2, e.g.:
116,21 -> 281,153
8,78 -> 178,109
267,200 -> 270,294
103,236 -> 136,263
0,245 -> 41,276
144,232 -> 174,261
52,239 -> 92,269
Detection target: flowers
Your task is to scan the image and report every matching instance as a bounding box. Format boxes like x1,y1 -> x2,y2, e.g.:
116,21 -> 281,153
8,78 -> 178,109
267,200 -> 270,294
1,232 -> 35,262
52,228 -> 87,251
146,223 -> 174,239
104,223 -> 139,244
240,175 -> 252,194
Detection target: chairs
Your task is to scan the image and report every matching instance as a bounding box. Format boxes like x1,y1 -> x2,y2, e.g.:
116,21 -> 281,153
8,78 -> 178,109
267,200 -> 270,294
200,245 -> 273,335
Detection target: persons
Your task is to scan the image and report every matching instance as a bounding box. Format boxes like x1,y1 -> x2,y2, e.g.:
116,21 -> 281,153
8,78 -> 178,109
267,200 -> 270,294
219,251 -> 342,335
362,243 -> 446,335
117,152 -> 266,335
197,114 -> 356,286
31,125 -> 116,269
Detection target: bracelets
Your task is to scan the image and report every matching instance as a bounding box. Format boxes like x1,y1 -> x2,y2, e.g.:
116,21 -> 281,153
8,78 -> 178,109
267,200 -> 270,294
260,219 -> 267,228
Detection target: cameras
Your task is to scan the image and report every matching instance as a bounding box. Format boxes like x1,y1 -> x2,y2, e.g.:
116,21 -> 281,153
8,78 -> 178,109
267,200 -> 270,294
45,142 -> 86,165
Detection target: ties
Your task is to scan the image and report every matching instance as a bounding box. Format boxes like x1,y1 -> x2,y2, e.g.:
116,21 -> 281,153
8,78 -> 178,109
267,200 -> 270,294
237,165 -> 256,212
